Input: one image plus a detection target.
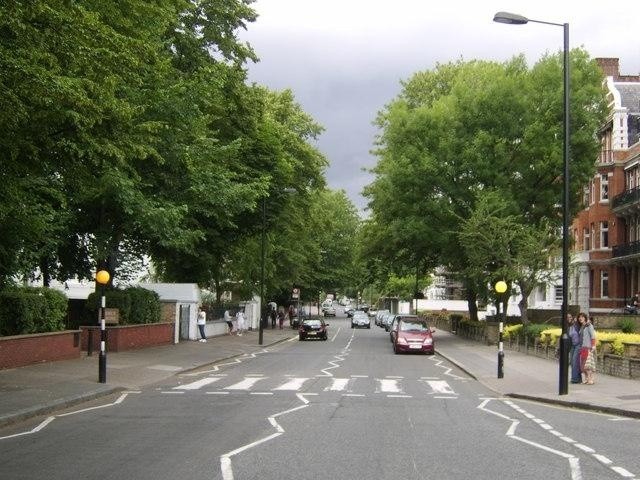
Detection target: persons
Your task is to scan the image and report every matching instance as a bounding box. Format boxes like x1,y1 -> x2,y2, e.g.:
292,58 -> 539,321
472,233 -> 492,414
288,305 -> 295,329
197,307 -> 208,343
277,310 -> 285,329
270,306 -> 277,329
236,307 -> 246,336
627,294 -> 640,315
224,308 -> 234,336
566,314 -> 584,383
577,312 -> 597,384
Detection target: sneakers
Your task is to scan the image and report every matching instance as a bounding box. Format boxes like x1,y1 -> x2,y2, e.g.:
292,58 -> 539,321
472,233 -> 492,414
236,332 -> 242,336
582,380 -> 593,385
229,332 -> 234,336
198,338 -> 206,343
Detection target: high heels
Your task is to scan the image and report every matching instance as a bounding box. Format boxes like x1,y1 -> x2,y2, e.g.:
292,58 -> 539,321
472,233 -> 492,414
570,376 -> 582,384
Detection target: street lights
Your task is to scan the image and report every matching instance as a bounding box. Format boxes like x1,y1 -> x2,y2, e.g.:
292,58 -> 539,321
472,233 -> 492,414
96,270 -> 113,385
257,188 -> 299,346
492,10 -> 575,397
494,279 -> 511,380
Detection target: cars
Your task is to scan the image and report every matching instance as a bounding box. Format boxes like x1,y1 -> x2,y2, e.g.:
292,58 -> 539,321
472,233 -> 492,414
389,316 -> 438,356
289,296 -> 422,343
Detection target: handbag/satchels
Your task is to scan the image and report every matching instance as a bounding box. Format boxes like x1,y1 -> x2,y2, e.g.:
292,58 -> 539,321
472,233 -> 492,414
583,350 -> 596,372
